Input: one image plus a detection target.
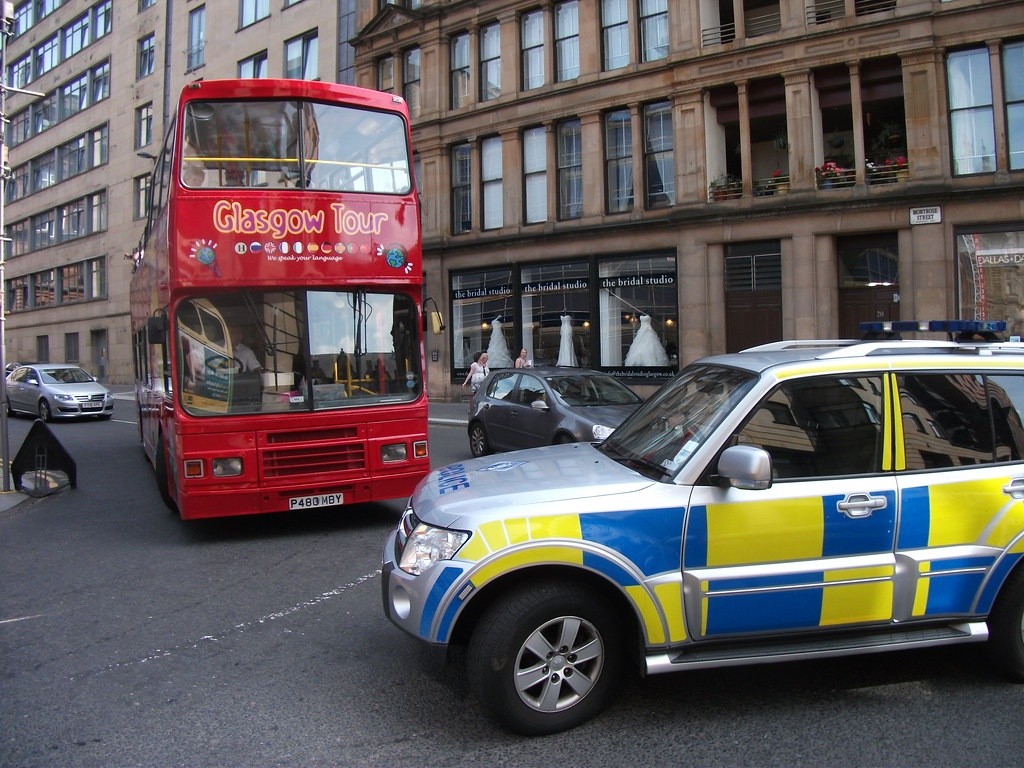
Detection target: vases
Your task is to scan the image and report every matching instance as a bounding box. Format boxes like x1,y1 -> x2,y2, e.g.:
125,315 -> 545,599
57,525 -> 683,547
776,180 -> 789,192
867,172 -> 881,183
896,168 -> 907,182
823,176 -> 835,189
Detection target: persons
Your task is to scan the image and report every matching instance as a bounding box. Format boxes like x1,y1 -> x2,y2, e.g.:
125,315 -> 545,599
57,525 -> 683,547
487,314 -> 515,367
183,165 -> 205,186
185,321 -> 263,386
223,161 -> 246,186
515,348 -> 531,368
555,312 -> 579,367
462,352 -> 490,392
624,309 -> 671,366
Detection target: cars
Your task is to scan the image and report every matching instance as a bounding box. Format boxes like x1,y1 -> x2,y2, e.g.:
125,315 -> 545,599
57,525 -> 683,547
5,361 -> 40,378
6,364 -> 115,423
467,367 -> 647,458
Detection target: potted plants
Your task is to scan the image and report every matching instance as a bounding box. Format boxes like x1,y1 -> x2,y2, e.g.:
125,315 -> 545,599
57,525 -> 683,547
709,178 -> 724,200
724,177 -> 741,199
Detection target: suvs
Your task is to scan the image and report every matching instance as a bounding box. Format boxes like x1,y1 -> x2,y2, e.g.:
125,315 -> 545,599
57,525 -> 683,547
383,320 -> 1024,738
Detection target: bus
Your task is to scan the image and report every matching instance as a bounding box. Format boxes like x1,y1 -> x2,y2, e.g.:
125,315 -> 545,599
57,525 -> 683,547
129,79 -> 446,521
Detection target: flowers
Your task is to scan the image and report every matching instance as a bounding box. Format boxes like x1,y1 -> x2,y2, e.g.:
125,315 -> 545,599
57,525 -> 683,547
815,162 -> 847,179
884,156 -> 907,172
768,170 -> 788,185
864,158 -> 878,172
